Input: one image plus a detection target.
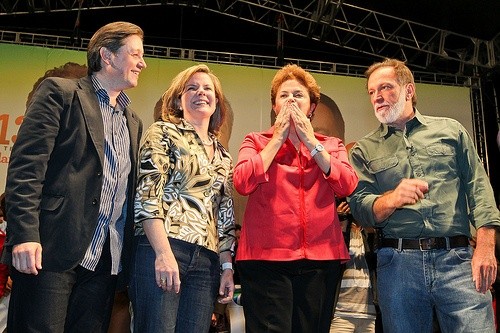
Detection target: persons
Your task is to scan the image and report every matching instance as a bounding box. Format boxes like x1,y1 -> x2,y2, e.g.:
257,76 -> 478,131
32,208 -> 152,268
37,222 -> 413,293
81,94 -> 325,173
330,222 -> 377,333
346,59 -> 500,333
3,22 -> 147,333
124,64 -> 236,333
271,92 -> 345,146
233,65 -> 360,333
235,224 -> 242,247
0,208 -> 13,333
154,88 -> 234,152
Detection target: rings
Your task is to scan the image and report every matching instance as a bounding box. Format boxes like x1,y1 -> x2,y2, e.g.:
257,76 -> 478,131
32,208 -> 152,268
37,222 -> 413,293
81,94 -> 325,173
160,278 -> 166,284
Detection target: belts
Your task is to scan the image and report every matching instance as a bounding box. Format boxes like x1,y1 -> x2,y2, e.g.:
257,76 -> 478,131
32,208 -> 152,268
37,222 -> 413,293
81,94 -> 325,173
381,236 -> 468,251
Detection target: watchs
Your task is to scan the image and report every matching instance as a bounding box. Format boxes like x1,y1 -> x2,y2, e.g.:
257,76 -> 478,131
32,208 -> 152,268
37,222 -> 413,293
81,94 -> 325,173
310,143 -> 324,157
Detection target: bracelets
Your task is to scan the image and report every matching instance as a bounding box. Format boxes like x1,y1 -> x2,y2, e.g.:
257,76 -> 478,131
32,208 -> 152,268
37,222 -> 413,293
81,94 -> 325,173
220,262 -> 235,274
